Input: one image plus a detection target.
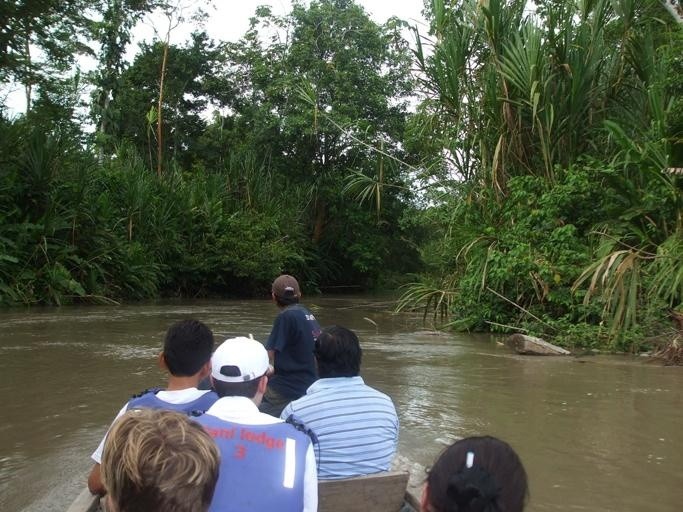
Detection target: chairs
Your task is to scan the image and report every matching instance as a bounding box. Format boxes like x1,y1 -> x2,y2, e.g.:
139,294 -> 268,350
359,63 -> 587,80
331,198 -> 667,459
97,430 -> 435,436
315,469 -> 410,512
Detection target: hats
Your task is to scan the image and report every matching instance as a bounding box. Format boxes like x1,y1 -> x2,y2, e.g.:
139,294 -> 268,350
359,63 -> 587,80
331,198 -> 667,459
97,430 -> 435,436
210,333 -> 270,385
270,274 -> 302,301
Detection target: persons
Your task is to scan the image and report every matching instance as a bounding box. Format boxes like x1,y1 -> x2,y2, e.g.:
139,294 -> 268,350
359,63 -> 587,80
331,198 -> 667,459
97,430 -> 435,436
257,271 -> 324,418
94,406 -> 225,511
415,430 -> 530,511
86,314 -> 225,496
280,325 -> 400,483
191,335 -> 325,512
